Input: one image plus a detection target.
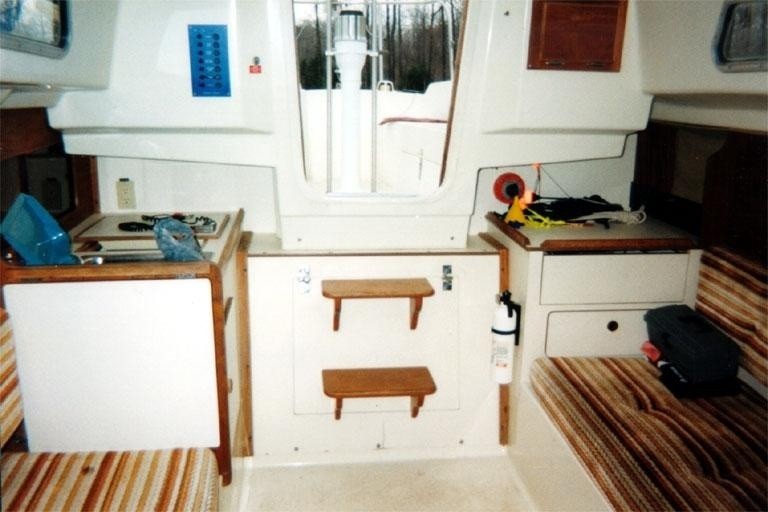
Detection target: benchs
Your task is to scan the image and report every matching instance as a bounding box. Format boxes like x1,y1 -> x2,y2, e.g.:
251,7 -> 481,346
520,252 -> 768,512
0,317 -> 230,512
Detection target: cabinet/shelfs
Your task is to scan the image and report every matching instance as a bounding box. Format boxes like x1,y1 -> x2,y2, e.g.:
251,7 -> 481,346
488,209 -> 710,358
5,214 -> 256,485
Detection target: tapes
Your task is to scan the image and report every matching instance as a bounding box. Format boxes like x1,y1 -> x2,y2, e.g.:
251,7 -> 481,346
493,172 -> 525,205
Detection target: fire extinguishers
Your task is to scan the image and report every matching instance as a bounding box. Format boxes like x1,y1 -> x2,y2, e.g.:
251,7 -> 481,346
488,288 -> 520,385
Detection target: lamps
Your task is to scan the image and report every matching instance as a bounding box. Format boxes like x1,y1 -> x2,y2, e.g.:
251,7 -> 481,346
326,0 -> 379,195
